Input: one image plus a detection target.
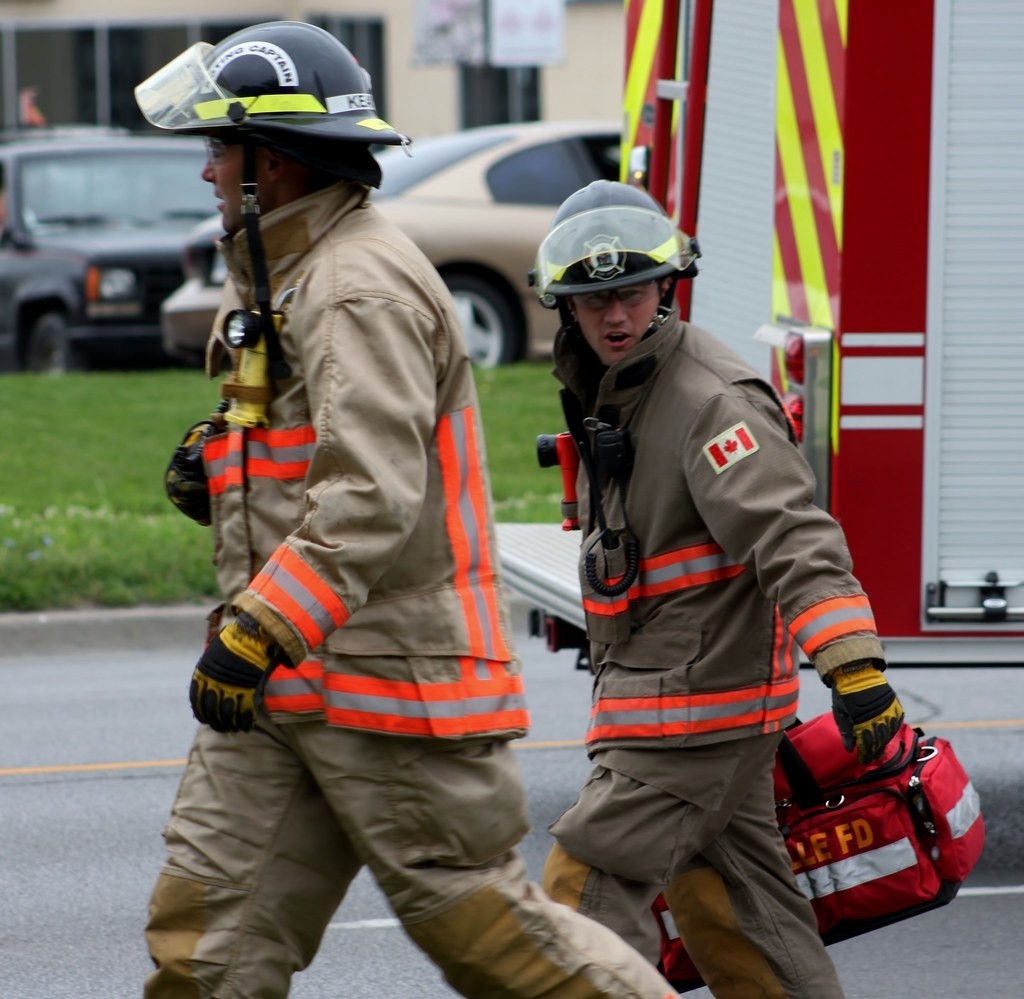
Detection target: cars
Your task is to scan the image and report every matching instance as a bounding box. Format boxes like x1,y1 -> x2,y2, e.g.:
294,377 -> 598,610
1,131 -> 221,377
160,117 -> 629,370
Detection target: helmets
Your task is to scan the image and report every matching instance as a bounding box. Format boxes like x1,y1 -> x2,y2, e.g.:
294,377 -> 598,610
528,177 -> 701,292
174,21 -> 413,146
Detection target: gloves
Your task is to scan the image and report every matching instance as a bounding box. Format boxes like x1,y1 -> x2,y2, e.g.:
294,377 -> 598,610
826,662 -> 904,763
164,422 -> 213,526
189,611 -> 285,734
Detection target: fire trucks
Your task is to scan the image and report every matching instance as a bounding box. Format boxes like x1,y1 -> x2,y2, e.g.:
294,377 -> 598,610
482,1 -> 1022,669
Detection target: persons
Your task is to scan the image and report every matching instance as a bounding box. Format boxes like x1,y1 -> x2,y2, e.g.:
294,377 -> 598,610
526,177 -> 906,998
131,20 -> 675,999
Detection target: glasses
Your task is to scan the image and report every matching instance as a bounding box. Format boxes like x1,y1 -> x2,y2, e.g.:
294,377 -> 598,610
197,135 -> 241,166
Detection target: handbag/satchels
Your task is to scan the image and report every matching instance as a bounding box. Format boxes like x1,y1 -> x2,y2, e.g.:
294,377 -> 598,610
650,711 -> 984,993
